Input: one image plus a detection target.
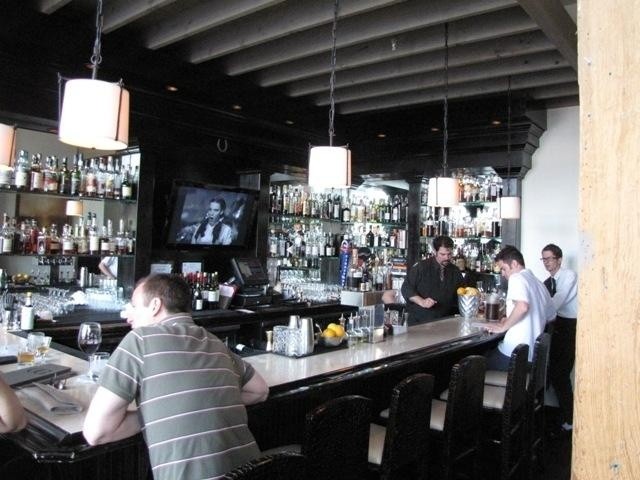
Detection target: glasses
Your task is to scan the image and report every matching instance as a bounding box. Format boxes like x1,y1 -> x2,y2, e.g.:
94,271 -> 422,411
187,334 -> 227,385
540,257 -> 556,261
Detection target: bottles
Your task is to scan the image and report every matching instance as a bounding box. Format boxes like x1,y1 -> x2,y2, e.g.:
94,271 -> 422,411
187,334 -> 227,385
32,257 -> 76,286
419,237 -> 502,275
419,204 -> 500,236
14,145 -> 139,205
338,312 -> 369,347
20,291 -> 36,332
421,176 -> 501,205
268,219 -> 405,258
267,184 -> 408,224
223,276 -> 237,286
267,258 -> 386,293
179,269 -> 221,312
383,307 -> 409,340
1,212 -> 135,255
477,280 -> 484,293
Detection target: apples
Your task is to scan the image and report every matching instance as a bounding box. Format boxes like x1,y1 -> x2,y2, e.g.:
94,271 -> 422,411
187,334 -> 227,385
457,287 -> 465,295
322,323 -> 345,337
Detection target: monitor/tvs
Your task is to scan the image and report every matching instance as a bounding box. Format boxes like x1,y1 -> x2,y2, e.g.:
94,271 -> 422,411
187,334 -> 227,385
160,179 -> 260,251
232,256 -> 269,297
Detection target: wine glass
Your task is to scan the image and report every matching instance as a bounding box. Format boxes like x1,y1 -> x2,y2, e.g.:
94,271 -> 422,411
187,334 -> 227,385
2,288 -> 124,322
76,323 -> 103,384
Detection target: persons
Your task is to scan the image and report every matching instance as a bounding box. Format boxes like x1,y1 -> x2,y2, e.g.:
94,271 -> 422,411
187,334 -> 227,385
401,236 -> 486,326
289,222 -> 304,240
485,244 -> 556,373
0,370 -> 29,438
542,243 -> 578,441
456,254 -> 469,278
82,272 -> 271,480
189,197 -> 231,246
99,256 -> 118,280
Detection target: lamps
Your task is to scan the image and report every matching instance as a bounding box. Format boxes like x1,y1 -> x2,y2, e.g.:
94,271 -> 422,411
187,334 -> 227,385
51,0 -> 130,153
428,15 -> 461,210
497,70 -> 523,223
308,1 -> 351,190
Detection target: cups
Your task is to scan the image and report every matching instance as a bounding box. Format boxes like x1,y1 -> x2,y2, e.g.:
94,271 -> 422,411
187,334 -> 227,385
1,165 -> 13,185
278,269 -> 341,303
17,332 -> 53,367
0,268 -> 9,287
79,266 -> 117,287
89,350 -> 110,379
272,325 -> 303,357
3,305 -> 19,332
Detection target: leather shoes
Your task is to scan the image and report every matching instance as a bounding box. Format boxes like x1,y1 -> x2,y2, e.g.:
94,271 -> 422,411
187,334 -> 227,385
550,423 -> 572,437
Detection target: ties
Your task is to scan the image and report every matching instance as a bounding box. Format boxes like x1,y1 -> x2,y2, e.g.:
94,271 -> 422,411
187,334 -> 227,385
551,278 -> 556,295
439,264 -> 446,282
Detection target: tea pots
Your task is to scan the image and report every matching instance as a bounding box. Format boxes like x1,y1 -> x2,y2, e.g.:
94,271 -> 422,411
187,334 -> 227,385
291,313 -> 324,354
485,294 -> 505,321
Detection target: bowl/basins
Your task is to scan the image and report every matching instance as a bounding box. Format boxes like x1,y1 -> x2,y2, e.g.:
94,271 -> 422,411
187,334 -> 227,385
12,273 -> 31,284
315,331 -> 348,347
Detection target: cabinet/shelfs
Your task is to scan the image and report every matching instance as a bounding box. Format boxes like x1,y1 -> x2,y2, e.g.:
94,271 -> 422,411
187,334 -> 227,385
1,130 -> 139,291
417,172 -> 503,276
269,179 -> 345,274
346,177 -> 416,272
